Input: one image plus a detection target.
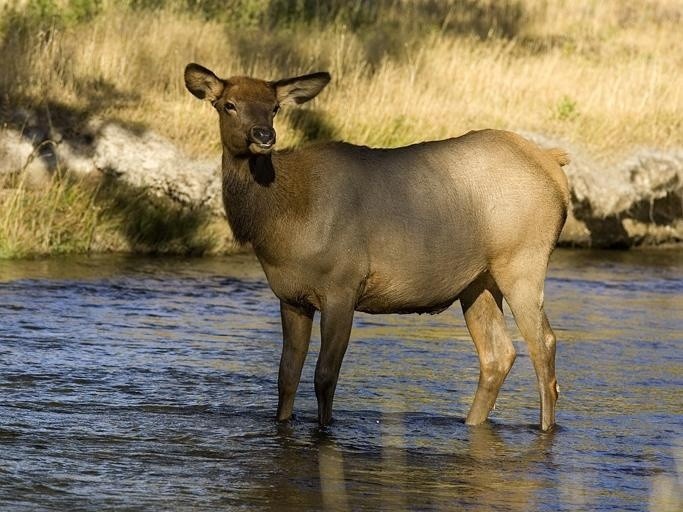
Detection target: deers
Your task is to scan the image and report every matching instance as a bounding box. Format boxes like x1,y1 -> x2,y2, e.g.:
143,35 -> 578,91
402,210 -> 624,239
183,62 -> 571,432
